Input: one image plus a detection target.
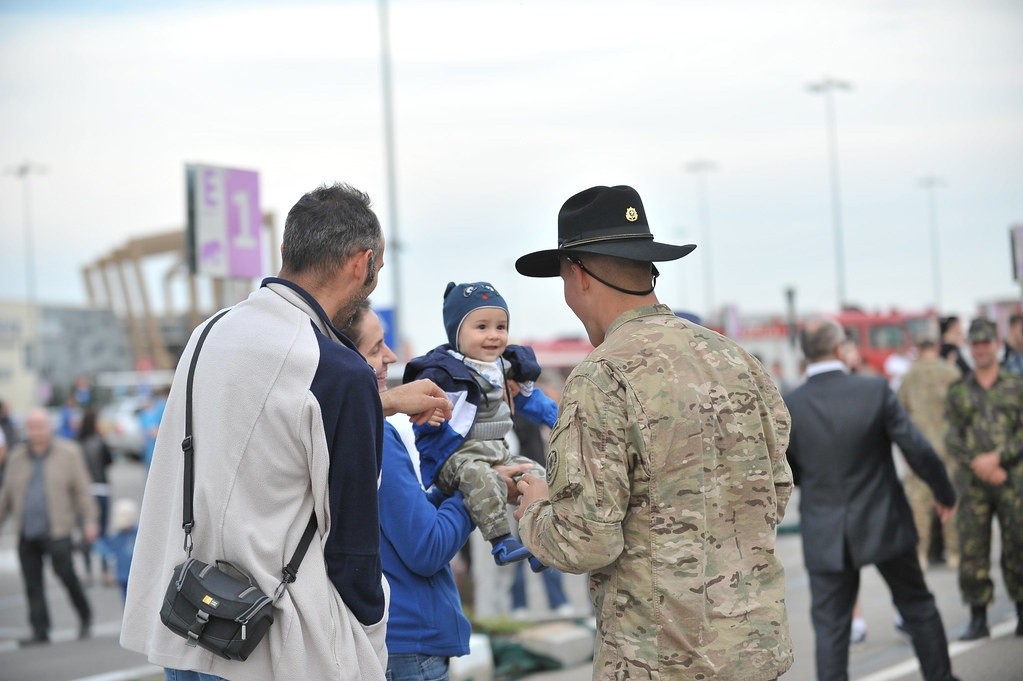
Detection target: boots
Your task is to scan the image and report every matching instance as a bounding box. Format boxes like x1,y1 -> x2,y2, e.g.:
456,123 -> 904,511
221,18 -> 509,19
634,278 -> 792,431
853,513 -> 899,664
959,604 -> 990,639
1015,601 -> 1023,636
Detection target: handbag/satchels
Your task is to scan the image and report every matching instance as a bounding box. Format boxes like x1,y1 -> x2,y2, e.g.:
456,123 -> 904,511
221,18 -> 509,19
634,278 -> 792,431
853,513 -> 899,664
159,560 -> 277,662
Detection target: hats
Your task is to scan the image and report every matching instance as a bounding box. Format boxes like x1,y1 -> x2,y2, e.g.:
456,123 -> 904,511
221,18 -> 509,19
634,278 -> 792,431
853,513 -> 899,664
443,282 -> 514,428
969,321 -> 997,343
515,185 -> 696,278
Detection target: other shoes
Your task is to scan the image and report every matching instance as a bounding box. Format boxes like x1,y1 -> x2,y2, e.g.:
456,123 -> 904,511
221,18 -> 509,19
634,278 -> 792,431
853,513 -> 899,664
492,537 -> 530,565
527,554 -> 549,572
78,615 -> 91,638
17,633 -> 49,646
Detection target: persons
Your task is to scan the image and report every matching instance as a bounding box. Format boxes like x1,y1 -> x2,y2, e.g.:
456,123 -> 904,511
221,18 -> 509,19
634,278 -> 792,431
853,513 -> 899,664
0,366 -> 165,649
119,187 -> 451,680
508,419 -> 577,617
402,281 -> 558,572
894,314 -> 1023,638
346,301 -> 534,680
515,186 -> 794,681
786,317 -> 961,681
